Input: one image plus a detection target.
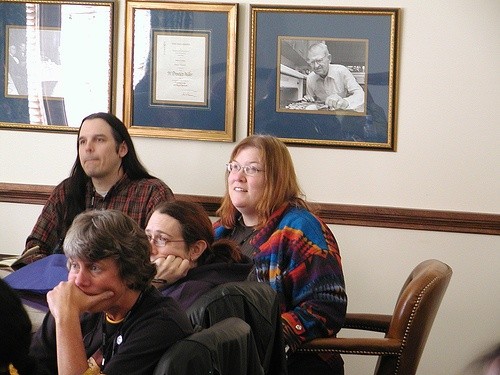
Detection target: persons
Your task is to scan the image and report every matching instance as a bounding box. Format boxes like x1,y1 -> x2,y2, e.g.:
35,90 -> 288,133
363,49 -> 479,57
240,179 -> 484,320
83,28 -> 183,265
22,112 -> 176,260
144,199 -> 253,312
212,134 -> 348,375
302,42 -> 365,111
27,208 -> 195,375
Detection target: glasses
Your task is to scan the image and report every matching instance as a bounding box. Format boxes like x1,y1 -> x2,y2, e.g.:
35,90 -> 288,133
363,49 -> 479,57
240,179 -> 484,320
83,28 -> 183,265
146,234 -> 190,247
305,54 -> 326,64
226,162 -> 266,176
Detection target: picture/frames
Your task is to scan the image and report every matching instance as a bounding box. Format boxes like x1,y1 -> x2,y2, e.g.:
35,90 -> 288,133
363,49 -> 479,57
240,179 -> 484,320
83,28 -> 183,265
0,0 -> 120,134
123,0 -> 238,143
248,4 -> 400,151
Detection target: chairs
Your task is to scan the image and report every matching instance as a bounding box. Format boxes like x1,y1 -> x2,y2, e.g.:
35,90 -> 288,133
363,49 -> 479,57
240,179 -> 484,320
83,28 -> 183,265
156,282 -> 285,375
295,259 -> 452,375
